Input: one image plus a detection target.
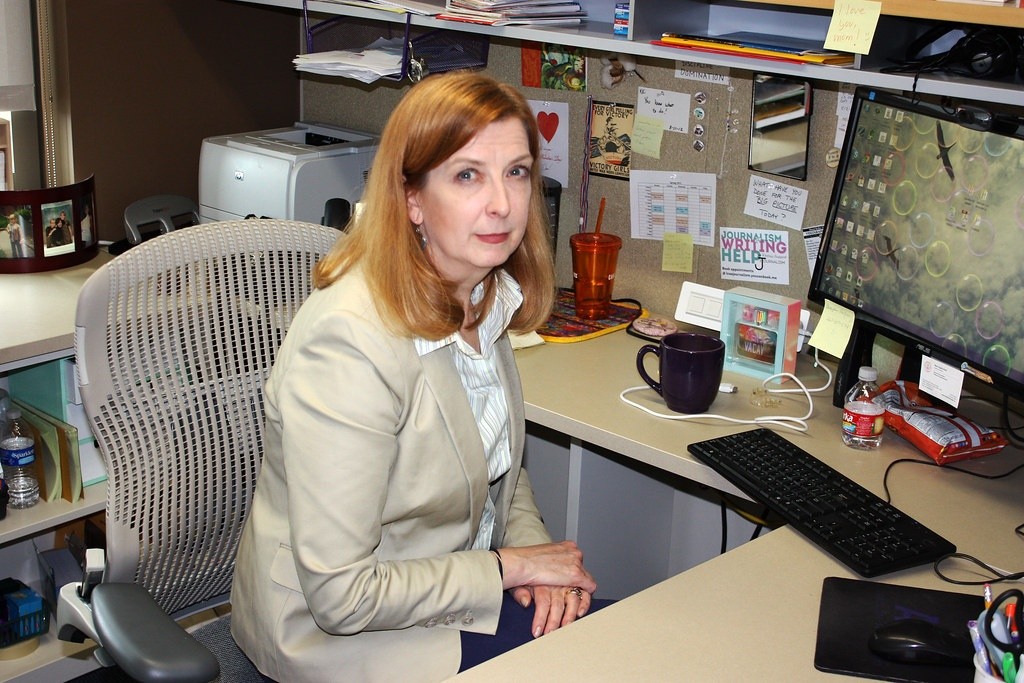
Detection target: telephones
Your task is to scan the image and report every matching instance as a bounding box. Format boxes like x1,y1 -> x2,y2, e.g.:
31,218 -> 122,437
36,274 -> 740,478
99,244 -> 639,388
320,198 -> 350,231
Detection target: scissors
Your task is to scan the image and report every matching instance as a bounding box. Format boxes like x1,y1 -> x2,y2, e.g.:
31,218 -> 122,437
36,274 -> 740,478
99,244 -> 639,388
984,589 -> 1024,673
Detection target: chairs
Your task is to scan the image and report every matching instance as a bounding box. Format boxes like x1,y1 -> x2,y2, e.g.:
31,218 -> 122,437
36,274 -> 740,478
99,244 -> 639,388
58,219 -> 346,683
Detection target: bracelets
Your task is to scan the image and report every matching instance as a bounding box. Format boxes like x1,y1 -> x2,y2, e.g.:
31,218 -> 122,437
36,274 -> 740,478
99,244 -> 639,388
489,547 -> 503,580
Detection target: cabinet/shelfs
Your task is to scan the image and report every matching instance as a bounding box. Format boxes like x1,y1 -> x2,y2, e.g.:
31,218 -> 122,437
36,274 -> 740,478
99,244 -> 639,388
8,335 -> 182,487
237,0 -> 1024,683
0,249 -> 219,683
0,118 -> 13,191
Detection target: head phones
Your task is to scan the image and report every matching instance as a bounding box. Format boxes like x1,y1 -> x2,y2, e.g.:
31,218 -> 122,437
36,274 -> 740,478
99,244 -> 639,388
902,19 -> 1024,83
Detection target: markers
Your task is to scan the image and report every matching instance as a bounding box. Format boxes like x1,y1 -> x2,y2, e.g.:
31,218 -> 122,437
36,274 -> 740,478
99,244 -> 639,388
968,584 -> 1016,683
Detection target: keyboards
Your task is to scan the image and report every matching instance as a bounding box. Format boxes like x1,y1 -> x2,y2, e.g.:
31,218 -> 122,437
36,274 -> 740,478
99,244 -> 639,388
687,427 -> 957,578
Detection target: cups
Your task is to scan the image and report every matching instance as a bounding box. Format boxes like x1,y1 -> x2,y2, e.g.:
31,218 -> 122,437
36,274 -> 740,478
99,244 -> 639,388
973,653 -> 1004,683
636,333 -> 725,415
570,232 -> 622,320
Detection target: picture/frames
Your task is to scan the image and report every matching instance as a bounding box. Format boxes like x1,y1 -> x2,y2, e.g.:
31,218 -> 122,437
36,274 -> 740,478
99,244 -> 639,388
0,173 -> 100,274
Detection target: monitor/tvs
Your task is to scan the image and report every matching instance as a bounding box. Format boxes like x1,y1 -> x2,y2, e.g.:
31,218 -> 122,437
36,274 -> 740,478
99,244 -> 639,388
808,87 -> 1024,405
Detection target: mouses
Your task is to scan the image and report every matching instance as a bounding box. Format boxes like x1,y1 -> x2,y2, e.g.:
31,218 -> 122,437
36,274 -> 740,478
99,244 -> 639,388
868,619 -> 982,668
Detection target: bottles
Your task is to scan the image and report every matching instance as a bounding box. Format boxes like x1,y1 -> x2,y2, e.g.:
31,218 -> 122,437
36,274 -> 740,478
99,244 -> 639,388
842,367 -> 885,451
0,409 -> 40,510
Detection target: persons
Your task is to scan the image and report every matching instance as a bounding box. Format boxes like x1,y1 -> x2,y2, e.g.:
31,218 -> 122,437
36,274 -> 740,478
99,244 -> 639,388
229,71 -> 601,683
45,210 -> 73,248
7,213 -> 23,258
82,205 -> 91,219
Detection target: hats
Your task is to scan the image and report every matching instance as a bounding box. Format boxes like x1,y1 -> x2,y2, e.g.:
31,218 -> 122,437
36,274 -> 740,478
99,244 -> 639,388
7,214 -> 16,219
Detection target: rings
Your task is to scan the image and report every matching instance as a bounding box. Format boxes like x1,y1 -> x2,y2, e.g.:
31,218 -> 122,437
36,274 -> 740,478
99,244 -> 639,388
565,587 -> 582,603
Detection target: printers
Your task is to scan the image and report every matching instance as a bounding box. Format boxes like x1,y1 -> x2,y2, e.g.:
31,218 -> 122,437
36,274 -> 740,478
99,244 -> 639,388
198,119 -> 382,231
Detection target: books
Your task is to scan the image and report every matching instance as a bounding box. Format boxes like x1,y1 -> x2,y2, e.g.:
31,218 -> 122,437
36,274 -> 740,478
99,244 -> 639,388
435,0 -> 589,27
